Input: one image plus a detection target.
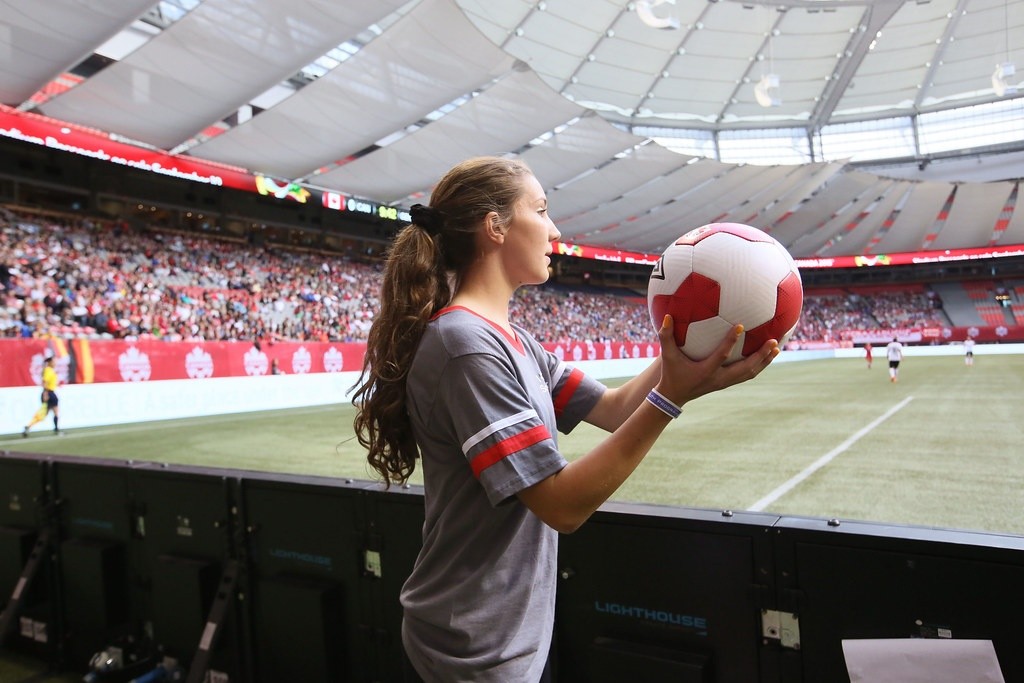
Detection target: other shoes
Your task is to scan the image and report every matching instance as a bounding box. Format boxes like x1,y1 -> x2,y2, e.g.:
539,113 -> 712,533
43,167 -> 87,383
55,428 -> 61,434
23,426 -> 29,438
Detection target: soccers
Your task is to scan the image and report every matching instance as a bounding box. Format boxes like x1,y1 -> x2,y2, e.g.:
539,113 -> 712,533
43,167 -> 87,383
648,222 -> 802,368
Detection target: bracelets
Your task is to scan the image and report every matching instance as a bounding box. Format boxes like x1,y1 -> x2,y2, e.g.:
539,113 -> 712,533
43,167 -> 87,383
645,387 -> 683,420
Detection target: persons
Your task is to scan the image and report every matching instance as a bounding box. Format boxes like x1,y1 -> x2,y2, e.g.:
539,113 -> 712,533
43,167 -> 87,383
21,355 -> 62,439
508,282 -> 660,343
963,335 -> 975,366
0,215 -> 385,343
788,287 -> 936,343
864,339 -> 873,368
350,156 -> 782,683
885,336 -> 903,384
